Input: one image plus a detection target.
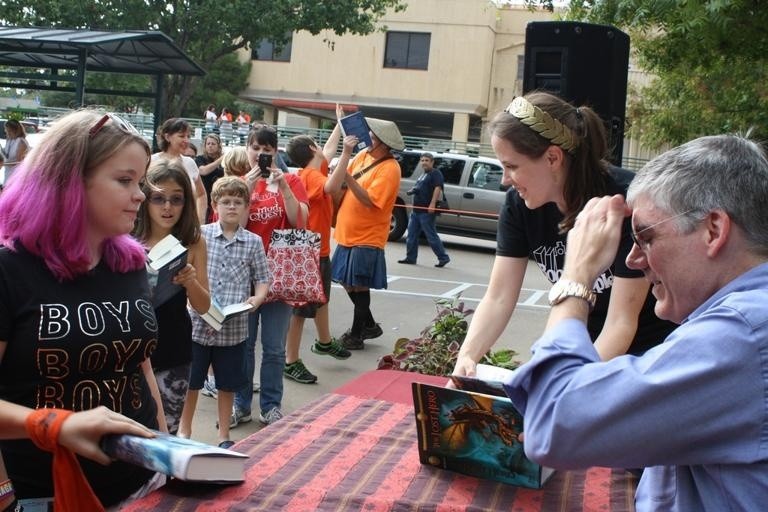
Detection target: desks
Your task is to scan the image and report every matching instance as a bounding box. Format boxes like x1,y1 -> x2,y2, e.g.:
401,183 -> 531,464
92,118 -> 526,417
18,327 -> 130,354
115,369 -> 637,512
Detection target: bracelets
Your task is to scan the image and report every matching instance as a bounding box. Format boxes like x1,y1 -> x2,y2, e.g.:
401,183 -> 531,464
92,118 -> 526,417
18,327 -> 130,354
25,409 -> 72,452
2,501 -> 23,512
0,479 -> 15,500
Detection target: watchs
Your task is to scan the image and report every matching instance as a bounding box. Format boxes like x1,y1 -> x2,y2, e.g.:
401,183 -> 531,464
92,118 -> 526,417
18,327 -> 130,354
548,279 -> 597,314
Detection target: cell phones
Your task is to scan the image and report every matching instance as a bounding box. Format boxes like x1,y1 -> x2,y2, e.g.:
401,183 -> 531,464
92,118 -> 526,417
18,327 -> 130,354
258,154 -> 272,178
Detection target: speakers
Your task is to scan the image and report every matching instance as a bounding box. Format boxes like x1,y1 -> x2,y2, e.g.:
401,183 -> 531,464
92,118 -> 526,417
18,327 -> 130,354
522,20 -> 631,167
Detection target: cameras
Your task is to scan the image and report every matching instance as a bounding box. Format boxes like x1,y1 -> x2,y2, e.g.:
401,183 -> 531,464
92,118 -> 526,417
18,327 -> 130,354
406,186 -> 420,196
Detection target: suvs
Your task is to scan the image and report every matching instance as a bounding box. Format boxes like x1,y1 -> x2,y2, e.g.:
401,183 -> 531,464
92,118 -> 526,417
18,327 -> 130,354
386,146 -> 512,244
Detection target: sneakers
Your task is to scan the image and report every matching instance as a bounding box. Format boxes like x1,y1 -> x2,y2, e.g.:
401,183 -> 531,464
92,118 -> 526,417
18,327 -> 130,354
284,358 -> 317,384
216,404 -> 252,431
311,337 -> 351,360
202,376 -> 219,400
339,331 -> 364,350
363,323 -> 383,340
259,407 -> 286,424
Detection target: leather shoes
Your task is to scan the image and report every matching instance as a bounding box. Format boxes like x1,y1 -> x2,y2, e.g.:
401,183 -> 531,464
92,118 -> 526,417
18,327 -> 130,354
398,258 -> 415,264
435,259 -> 450,268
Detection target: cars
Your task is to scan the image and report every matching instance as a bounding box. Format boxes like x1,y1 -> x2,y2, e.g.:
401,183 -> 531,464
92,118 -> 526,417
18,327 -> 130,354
0,119 -> 38,144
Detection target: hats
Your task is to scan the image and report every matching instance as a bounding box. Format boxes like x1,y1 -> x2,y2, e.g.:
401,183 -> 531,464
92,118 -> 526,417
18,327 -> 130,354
365,117 -> 405,152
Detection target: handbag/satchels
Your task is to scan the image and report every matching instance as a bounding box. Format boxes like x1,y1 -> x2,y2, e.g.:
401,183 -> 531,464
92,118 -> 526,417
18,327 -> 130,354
434,199 -> 450,213
263,227 -> 327,307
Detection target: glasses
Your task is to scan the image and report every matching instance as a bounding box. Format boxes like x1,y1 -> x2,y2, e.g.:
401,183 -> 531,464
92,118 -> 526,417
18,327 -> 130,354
148,193 -> 185,206
629,205 -> 699,250
88,112 -> 142,139
254,123 -> 277,133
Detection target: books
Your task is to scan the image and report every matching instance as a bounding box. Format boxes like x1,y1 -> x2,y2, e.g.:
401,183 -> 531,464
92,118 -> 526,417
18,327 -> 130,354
99,428 -> 250,486
450,361 -> 514,398
411,381 -> 558,491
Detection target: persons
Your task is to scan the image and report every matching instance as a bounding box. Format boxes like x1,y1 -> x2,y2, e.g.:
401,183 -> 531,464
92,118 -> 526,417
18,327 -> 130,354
399,153 -> 449,267
502,133 -> 768,511
1,106 -> 171,512
0,399 -> 156,463
129,105 -> 404,442
0,119 -> 31,185
444,91 -> 681,391
